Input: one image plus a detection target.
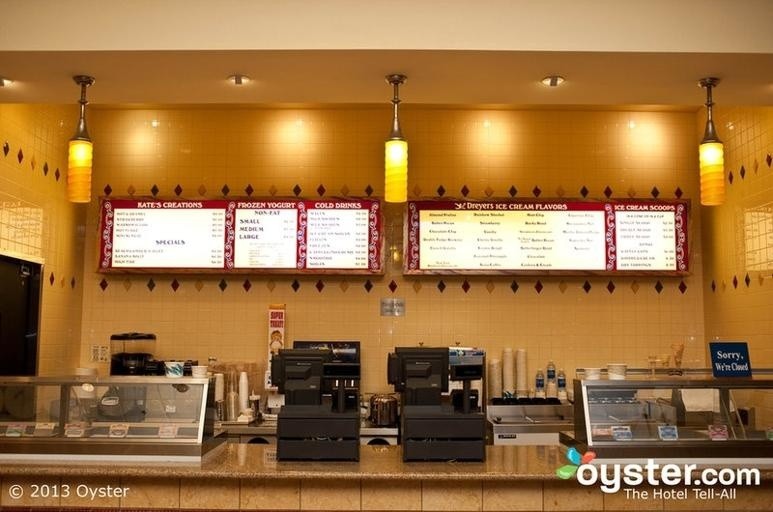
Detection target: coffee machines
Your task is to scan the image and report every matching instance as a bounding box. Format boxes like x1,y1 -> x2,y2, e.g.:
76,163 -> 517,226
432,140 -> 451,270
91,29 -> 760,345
108,330 -> 196,378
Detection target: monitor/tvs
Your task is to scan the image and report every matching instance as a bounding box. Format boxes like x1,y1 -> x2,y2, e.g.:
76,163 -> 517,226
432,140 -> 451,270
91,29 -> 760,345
271,349 -> 332,405
386,347 -> 449,405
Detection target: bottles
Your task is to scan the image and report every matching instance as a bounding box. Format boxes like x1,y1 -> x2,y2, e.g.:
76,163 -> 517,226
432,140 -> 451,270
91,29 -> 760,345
536,362 -> 567,404
206,355 -> 217,406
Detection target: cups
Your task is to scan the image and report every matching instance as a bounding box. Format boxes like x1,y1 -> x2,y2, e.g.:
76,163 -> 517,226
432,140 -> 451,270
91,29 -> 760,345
605,363 -> 627,379
162,360 -> 184,375
489,343 -> 529,397
214,370 -> 259,425
193,365 -> 206,379
368,391 -> 397,425
583,368 -> 602,379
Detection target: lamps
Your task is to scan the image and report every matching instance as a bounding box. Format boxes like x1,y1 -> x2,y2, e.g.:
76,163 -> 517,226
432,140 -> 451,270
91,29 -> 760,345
380,74 -> 410,205
691,75 -> 729,206
64,74 -> 99,205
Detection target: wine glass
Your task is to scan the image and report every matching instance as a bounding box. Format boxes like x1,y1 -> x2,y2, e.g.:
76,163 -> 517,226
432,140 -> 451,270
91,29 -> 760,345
642,340 -> 684,380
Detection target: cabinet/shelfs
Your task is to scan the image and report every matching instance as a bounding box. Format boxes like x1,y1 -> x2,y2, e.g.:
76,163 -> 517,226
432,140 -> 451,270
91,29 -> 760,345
0,373 -> 230,468
554,376 -> 773,478
